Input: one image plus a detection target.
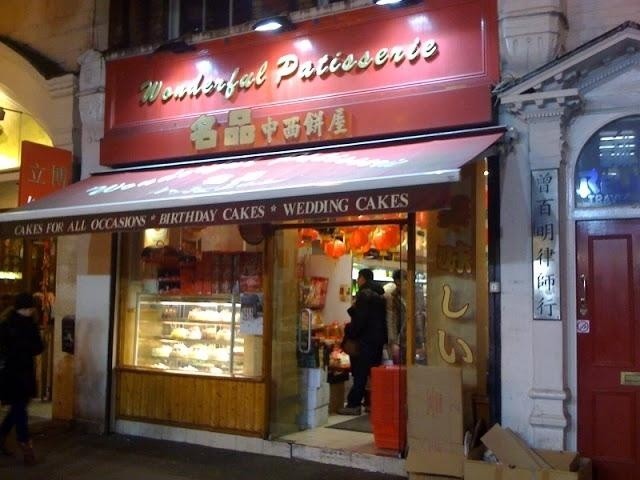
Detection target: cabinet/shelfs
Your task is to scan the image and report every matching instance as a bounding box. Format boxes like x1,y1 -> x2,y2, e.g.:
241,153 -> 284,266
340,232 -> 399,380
136,292 -> 245,377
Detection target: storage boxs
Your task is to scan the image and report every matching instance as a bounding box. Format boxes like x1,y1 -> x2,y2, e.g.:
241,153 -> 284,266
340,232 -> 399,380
465,443 -> 592,480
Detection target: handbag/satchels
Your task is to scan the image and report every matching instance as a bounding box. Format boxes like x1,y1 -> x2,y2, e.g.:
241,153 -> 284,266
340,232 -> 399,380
340,326 -> 359,354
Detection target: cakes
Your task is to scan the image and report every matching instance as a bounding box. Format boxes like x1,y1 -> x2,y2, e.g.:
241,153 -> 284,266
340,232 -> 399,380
150,307 -> 245,377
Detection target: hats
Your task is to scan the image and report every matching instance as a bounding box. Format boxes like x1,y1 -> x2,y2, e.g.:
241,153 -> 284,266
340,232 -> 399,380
15,292 -> 36,309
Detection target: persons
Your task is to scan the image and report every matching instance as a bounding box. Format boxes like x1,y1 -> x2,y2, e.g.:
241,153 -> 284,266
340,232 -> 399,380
0,292 -> 46,464
386,269 -> 426,366
336,269 -> 388,415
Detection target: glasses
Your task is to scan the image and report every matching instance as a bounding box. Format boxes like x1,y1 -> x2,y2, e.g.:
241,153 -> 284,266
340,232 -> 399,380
393,280 -> 408,288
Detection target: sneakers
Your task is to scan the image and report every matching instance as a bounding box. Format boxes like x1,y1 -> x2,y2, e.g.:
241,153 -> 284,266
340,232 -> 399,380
21,441 -> 36,464
337,404 -> 362,415
0,428 -> 16,458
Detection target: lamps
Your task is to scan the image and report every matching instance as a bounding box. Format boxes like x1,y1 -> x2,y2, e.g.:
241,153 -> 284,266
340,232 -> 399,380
252,16 -> 296,33
373,0 -> 423,9
148,40 -> 196,54
